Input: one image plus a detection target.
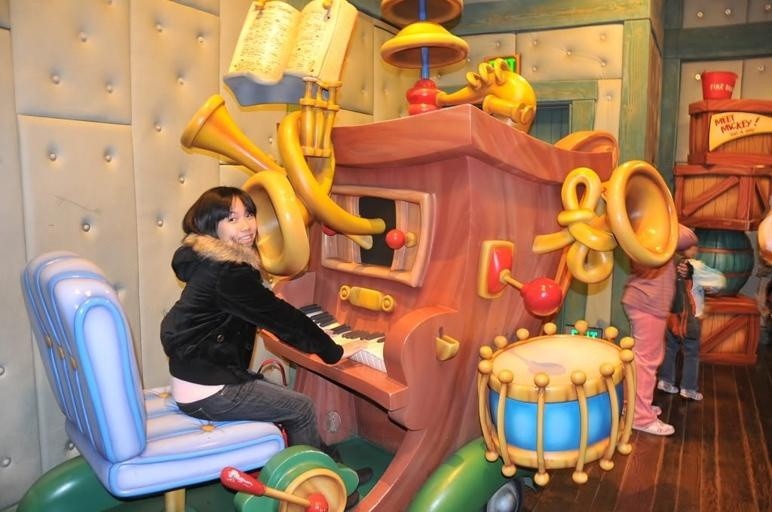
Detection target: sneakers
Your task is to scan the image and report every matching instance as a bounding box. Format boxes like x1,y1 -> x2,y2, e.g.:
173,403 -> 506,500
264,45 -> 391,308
633,418 -> 675,436
657,379 -> 679,394
622,405 -> 662,415
680,389 -> 703,401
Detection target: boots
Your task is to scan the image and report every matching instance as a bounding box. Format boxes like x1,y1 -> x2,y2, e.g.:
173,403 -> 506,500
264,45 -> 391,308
346,491 -> 359,508
329,447 -> 373,484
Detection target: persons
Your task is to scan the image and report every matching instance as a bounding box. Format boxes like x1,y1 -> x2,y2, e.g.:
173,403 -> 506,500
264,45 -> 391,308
161,185 -> 374,512
657,258 -> 727,400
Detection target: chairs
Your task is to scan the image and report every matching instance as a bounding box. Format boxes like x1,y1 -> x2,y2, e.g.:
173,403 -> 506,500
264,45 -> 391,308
20,252 -> 283,512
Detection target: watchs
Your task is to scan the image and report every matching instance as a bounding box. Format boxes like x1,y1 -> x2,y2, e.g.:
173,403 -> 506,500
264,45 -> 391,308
622,223 -> 699,435
